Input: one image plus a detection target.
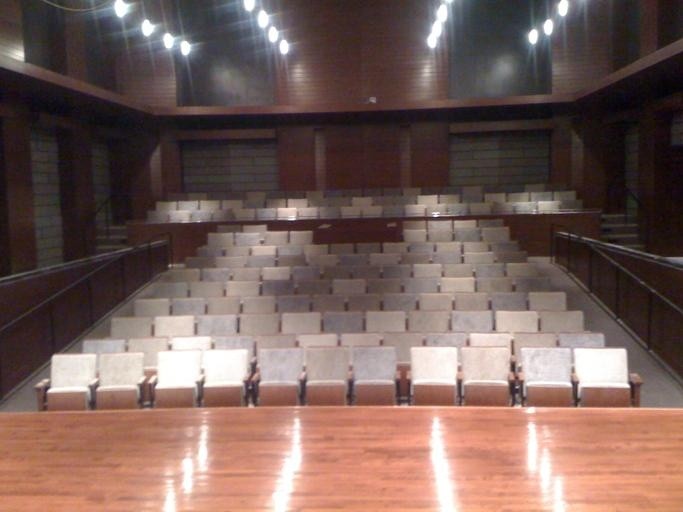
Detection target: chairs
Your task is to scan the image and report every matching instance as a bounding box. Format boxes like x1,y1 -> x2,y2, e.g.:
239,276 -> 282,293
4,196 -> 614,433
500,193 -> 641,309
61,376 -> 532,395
47,295 -> 274,408
453,291 -> 630,407
255,293 -> 458,404
159,219 -> 551,291
145,182 -> 578,222
